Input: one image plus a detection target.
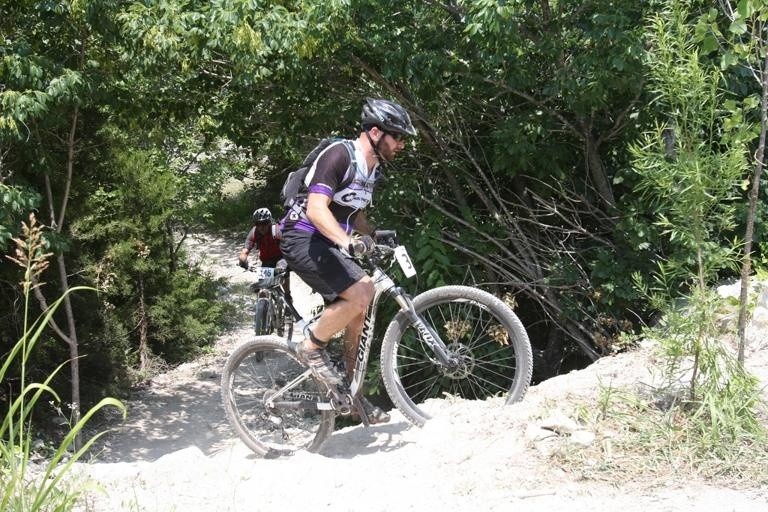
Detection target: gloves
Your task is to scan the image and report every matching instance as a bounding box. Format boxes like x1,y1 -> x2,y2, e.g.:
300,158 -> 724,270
276,259 -> 288,273
355,235 -> 376,255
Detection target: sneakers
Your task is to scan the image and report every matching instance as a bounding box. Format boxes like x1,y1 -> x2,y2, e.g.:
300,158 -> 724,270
294,341 -> 342,384
350,394 -> 390,422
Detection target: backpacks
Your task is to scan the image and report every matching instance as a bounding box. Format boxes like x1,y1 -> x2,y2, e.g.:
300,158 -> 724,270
278,135 -> 358,209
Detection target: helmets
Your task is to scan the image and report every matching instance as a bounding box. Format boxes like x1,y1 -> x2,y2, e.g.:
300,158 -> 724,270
361,97 -> 418,136
253,207 -> 273,223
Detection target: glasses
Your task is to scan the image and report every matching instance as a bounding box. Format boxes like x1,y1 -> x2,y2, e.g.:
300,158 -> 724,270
385,130 -> 404,141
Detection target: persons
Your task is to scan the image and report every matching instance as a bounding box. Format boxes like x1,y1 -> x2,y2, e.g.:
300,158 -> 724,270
239,208 -> 296,317
279,99 -> 417,424
